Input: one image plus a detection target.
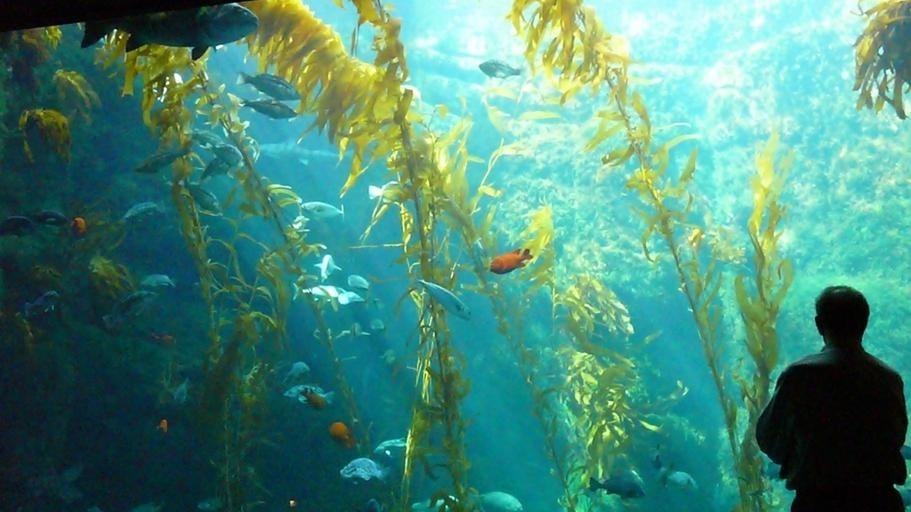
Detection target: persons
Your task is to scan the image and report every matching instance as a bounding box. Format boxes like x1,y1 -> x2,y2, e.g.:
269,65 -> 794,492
754,284 -> 910,510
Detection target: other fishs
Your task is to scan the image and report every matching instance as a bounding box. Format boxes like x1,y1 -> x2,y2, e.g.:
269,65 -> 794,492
0,59 -> 434,512
